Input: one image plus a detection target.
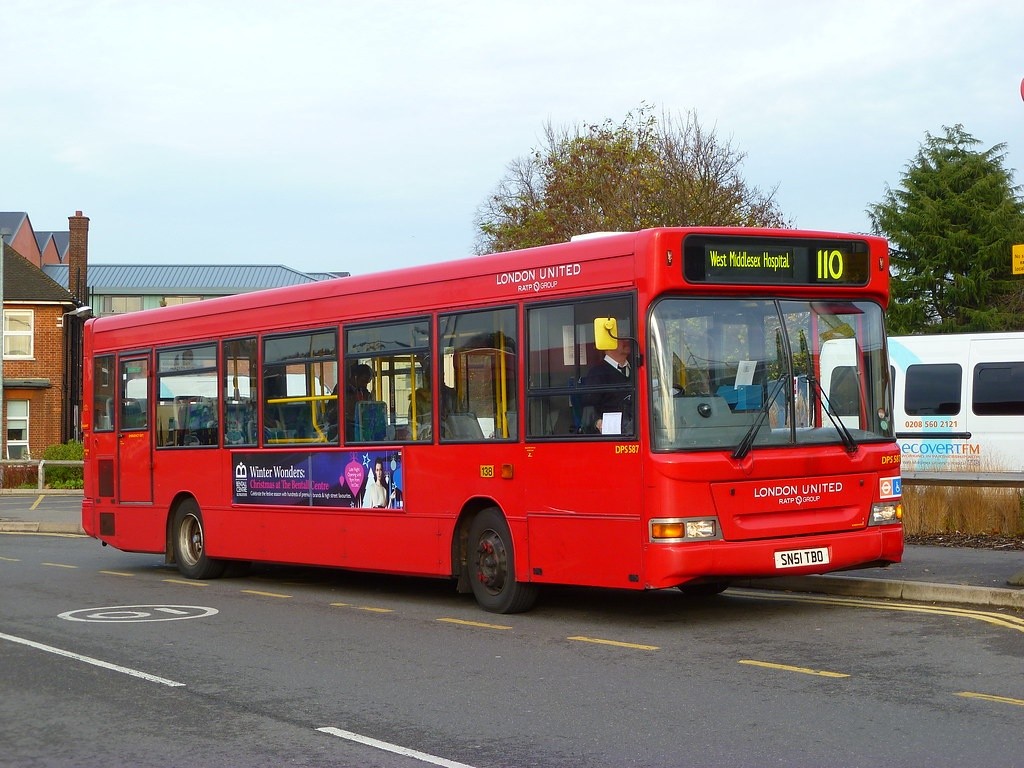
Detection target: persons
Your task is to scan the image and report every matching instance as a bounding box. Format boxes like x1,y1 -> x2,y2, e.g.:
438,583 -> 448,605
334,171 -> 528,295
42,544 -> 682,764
575,324 -> 633,434
370,458 -> 395,509
406,357 -> 457,440
326,364 -> 375,443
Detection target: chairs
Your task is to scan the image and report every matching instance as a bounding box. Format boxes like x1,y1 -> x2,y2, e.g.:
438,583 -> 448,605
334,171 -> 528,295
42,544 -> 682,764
278,393 -> 322,442
175,396 -> 214,445
215,396 -> 255,443
105,396 -> 146,429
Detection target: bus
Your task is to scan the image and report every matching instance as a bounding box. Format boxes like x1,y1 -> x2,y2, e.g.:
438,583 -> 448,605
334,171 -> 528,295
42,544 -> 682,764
80,224 -> 904,613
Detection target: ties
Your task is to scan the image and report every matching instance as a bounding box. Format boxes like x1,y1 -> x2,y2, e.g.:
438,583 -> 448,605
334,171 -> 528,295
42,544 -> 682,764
618,364 -> 627,377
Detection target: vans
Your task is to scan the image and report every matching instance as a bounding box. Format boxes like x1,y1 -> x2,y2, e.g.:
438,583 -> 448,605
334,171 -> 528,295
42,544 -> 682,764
819,332 -> 1024,472
